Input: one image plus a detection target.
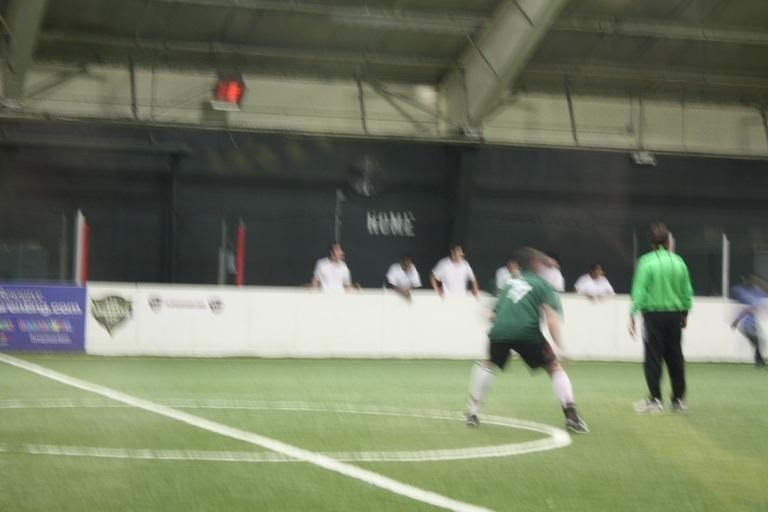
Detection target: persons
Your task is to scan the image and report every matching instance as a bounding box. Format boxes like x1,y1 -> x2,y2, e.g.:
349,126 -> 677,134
428,244 -> 480,302
539,256 -> 565,291
464,244 -> 587,432
493,257 -> 521,297
573,259 -> 614,298
626,219 -> 693,413
382,250 -> 422,305
727,274 -> 766,367
308,240 -> 359,292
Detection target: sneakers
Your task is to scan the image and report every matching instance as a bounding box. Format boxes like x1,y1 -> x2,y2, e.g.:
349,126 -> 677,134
633,396 -> 665,414
565,416 -> 588,434
466,414 -> 479,427
